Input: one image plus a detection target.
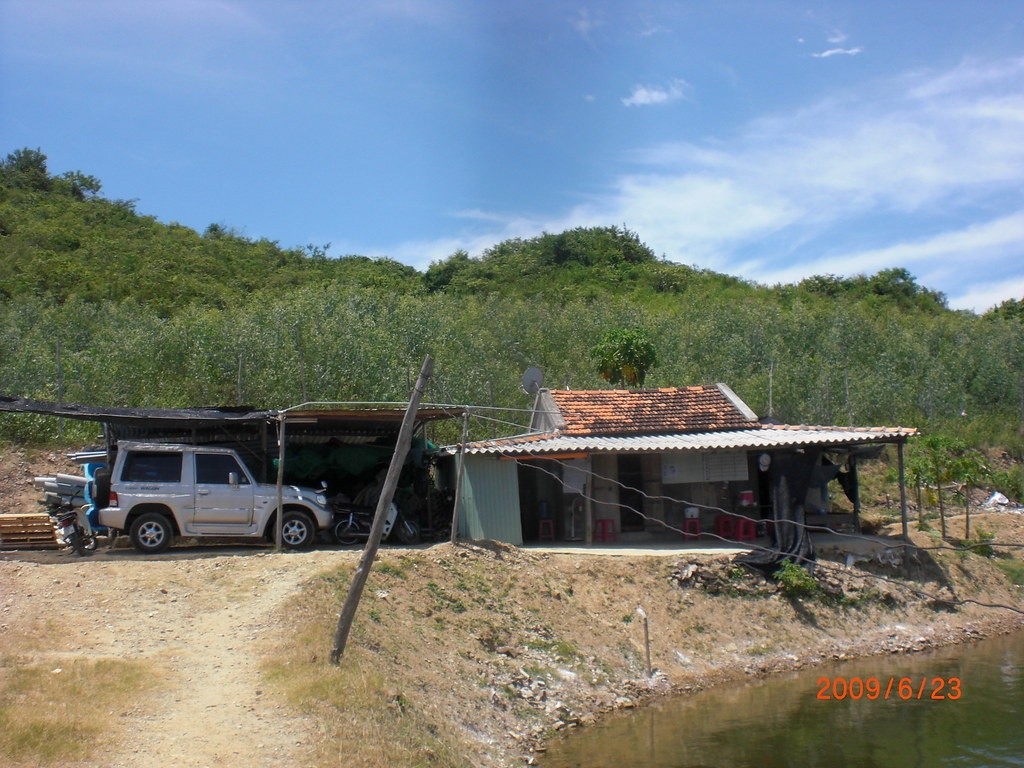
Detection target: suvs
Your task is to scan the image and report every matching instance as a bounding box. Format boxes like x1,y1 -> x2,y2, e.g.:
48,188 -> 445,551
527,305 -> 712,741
91,438 -> 335,550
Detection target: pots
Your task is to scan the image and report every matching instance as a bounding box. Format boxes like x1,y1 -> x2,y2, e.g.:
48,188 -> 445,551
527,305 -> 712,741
684,506 -> 699,518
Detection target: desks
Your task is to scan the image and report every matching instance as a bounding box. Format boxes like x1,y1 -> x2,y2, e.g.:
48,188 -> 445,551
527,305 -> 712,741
806,512 -> 855,529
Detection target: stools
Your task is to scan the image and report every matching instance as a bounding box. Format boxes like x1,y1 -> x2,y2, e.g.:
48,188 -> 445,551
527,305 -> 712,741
714,515 -> 735,538
592,518 -> 619,545
735,517 -> 756,542
538,518 -> 556,542
682,519 -> 703,542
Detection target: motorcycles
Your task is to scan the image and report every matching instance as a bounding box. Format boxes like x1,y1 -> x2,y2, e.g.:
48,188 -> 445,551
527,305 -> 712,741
45,489 -> 99,557
335,496 -> 420,547
